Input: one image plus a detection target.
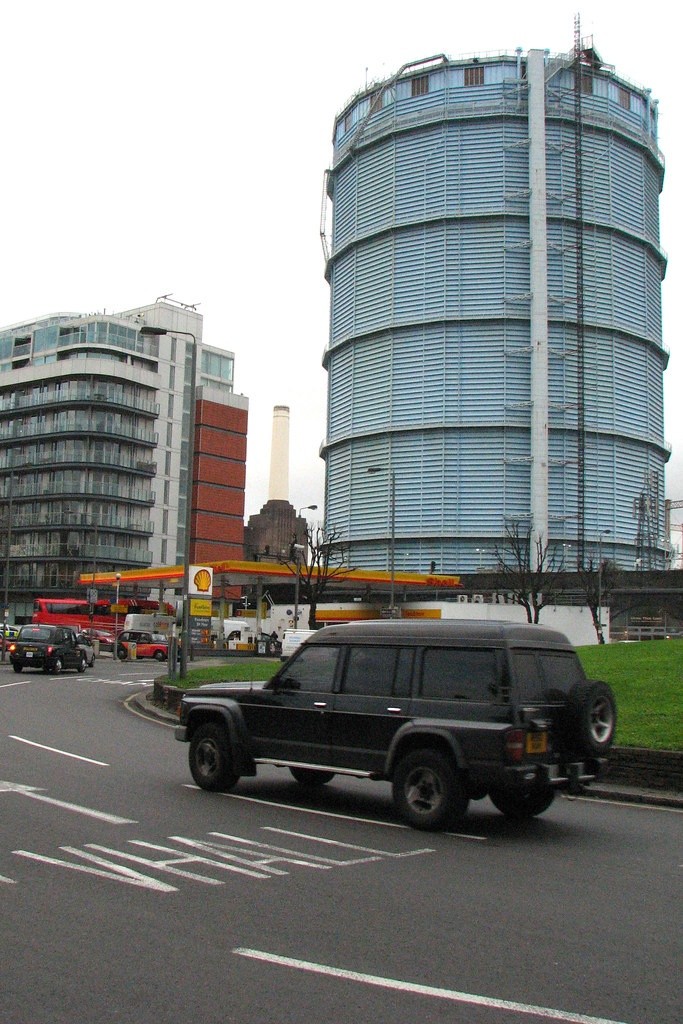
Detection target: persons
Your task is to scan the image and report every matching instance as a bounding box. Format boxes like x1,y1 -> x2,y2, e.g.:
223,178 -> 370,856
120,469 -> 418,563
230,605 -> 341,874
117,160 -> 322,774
269,631 -> 278,641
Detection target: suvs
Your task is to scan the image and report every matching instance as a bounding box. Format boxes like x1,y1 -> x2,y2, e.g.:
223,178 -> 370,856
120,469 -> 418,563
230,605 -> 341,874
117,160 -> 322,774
172,618 -> 620,830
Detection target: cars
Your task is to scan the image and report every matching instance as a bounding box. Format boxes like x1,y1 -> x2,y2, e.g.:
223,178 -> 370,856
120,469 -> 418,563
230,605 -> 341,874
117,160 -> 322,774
0,624 -> 20,640
80,627 -> 117,652
117,629 -> 181,663
65,633 -> 95,668
9,623 -> 87,676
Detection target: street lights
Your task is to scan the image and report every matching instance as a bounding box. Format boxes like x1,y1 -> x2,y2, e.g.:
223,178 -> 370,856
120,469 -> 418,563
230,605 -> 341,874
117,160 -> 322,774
598,529 -> 612,644
139,326 -> 197,679
64,510 -> 99,640
298,505 -> 318,545
368,468 -> 395,609
114,573 -> 121,659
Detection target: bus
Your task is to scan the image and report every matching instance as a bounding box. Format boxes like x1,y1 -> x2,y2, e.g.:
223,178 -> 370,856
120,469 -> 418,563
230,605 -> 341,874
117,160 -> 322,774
32,598 -> 176,644
